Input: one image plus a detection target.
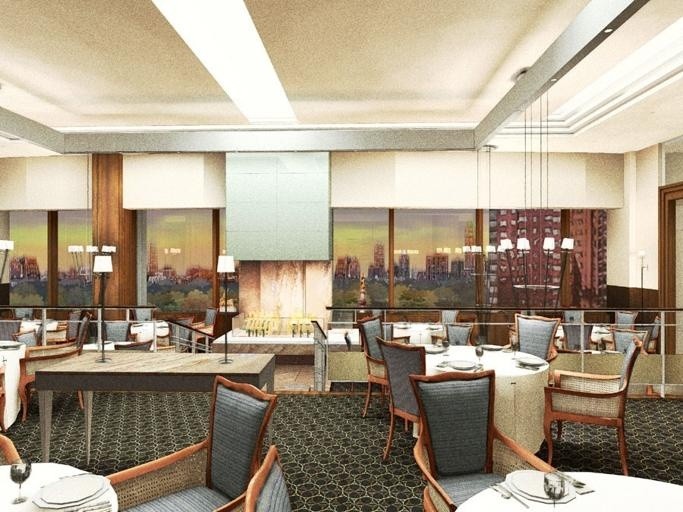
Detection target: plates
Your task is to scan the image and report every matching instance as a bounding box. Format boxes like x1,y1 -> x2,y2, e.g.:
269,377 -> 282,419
503,472 -> 575,504
30,472 -> 110,510
411,333 -> 545,380
510,469 -> 568,497
40,475 -> 102,503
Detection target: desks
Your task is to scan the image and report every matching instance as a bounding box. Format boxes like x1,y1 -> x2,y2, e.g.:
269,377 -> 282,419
33,352 -> 276,467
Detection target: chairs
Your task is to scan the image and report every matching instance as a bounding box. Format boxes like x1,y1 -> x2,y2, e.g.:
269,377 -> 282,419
509,313 -> 560,362
359,316 -> 408,430
0,433 -> 21,464
244,446 -> 290,510
0,307 -> 217,433
106,374 -> 278,512
541,334 -> 643,472
409,369 -> 557,511
375,335 -> 426,460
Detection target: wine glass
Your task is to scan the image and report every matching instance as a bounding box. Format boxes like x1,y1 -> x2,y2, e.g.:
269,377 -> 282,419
11,458 -> 30,504
544,472 -> 564,504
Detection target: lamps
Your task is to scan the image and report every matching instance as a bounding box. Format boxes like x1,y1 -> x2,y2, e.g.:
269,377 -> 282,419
67,154 -> 115,281
502,68 -> 575,314
92,256 -> 111,362
216,255 -> 234,365
